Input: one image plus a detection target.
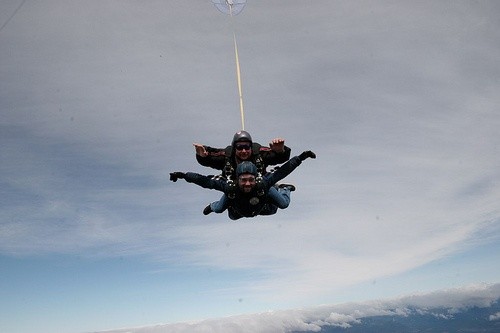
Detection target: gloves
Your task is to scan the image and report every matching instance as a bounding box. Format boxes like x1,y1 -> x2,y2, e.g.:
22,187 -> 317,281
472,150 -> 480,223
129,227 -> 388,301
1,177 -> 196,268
170,172 -> 185,182
298,151 -> 316,161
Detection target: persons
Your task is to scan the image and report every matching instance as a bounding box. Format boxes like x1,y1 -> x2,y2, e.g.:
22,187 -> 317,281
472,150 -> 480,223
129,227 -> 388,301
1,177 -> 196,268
170,151 -> 315,220
193,130 -> 296,215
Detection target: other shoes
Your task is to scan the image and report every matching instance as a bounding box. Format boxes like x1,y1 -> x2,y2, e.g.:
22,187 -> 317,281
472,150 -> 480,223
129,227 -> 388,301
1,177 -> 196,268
279,184 -> 295,191
203,205 -> 213,215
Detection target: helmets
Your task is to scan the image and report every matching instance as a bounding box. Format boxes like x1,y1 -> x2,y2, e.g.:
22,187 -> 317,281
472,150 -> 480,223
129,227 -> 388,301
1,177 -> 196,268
236,161 -> 257,176
232,130 -> 252,145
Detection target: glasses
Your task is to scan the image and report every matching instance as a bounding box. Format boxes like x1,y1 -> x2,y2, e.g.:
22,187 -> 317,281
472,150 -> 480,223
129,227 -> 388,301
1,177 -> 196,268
235,144 -> 251,150
238,177 -> 257,183
